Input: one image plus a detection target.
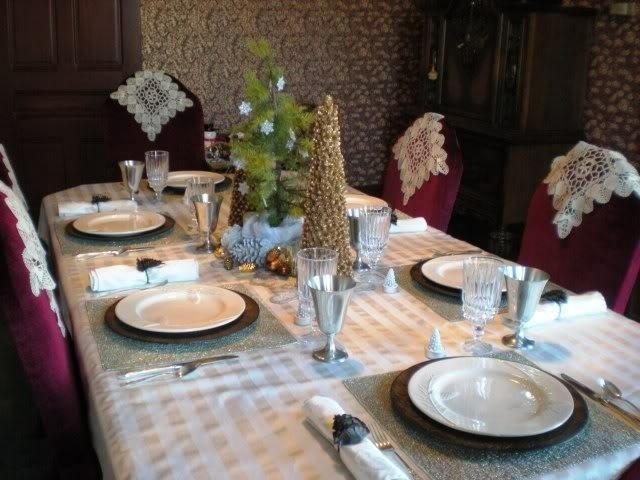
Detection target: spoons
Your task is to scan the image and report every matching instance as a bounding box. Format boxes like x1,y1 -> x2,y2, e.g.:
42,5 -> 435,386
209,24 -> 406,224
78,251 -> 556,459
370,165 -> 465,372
602,379 -> 639,414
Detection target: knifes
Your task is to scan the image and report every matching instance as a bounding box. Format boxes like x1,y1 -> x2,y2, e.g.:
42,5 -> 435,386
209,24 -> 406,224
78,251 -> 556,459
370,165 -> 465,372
560,372 -> 640,427
121,354 -> 239,377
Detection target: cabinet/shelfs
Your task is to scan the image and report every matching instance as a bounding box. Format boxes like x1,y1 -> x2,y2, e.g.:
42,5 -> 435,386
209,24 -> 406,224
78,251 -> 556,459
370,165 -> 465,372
417,1 -> 597,237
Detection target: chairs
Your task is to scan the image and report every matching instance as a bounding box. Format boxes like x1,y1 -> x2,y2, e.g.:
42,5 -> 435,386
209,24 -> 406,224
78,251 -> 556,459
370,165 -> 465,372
517,141 -> 640,313
108,70 -> 204,183
0,143 -> 86,442
381,109 -> 463,232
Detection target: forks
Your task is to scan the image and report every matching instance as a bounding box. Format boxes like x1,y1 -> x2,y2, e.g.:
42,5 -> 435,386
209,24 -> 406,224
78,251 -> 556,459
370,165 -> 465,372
119,357 -> 206,387
367,421 -> 423,480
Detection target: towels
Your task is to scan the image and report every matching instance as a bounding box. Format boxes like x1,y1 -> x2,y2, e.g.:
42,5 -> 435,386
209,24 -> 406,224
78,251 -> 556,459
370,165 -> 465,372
390,216 -> 428,236
56,198 -> 137,216
88,258 -> 200,292
304,396 -> 405,478
534,290 -> 606,327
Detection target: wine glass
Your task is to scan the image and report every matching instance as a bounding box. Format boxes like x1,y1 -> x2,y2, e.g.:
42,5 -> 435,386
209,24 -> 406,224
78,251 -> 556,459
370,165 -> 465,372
461,254 -> 505,355
306,275 -> 356,362
348,207 -> 371,272
119,159 -> 145,205
144,150 -> 170,206
296,247 -> 340,342
502,266 -> 552,350
189,193 -> 224,252
358,207 -> 391,283
185,175 -> 216,231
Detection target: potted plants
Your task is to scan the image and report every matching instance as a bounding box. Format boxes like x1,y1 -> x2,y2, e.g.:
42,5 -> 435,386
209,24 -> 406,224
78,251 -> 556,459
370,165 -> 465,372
214,38 -> 317,277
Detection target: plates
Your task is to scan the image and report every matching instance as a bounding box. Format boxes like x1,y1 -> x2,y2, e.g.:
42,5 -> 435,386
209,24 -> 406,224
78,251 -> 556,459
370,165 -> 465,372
114,283 -> 246,334
165,170 -> 225,189
421,254 -> 520,292
408,355 -> 575,439
72,210 -> 166,236
